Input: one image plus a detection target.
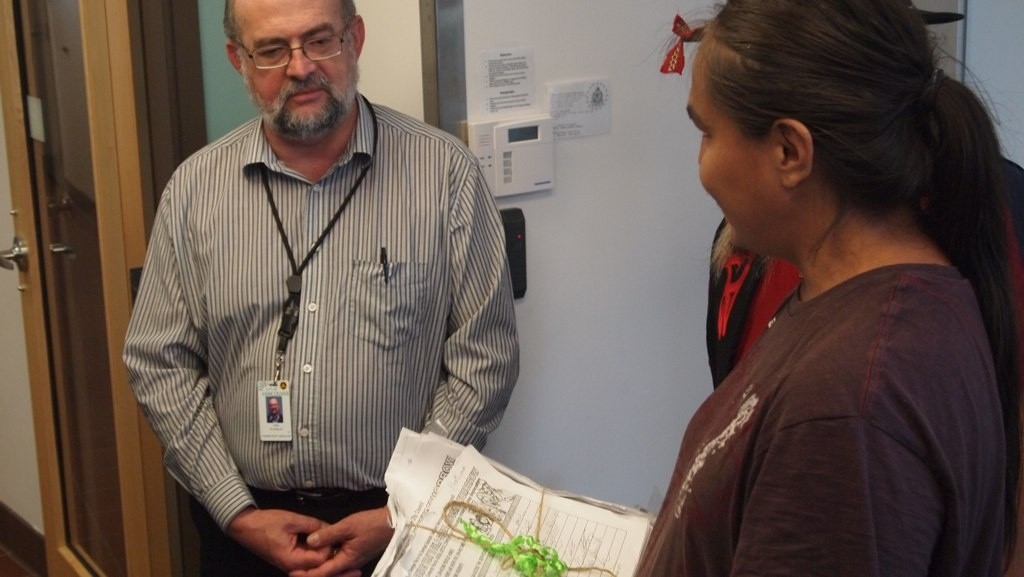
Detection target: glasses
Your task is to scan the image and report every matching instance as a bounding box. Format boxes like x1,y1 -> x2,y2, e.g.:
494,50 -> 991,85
235,20 -> 352,71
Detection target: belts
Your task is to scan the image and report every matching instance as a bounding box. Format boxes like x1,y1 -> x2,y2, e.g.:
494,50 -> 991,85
251,488 -> 387,511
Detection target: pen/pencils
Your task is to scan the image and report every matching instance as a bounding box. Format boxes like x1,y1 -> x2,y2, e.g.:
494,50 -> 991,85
378,246 -> 390,283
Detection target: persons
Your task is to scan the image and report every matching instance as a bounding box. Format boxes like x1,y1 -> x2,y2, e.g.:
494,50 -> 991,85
633,0 -> 1024,577
122,0 -> 518,577
266,397 -> 283,423
